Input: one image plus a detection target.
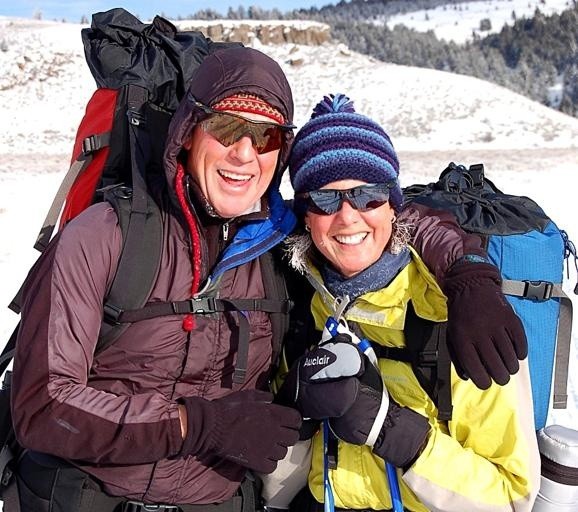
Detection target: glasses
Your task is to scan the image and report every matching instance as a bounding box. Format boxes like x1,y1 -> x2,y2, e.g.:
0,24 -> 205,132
293,180 -> 398,215
184,90 -> 298,155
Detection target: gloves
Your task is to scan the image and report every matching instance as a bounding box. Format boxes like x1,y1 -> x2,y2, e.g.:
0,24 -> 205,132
273,333 -> 433,470
437,259 -> 528,392
165,386 -> 304,478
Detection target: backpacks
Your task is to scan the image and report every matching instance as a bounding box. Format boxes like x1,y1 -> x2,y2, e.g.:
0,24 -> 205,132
293,160 -> 578,435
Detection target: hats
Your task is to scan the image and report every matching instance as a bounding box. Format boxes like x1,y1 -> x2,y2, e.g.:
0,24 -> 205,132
204,90 -> 290,125
287,91 -> 404,216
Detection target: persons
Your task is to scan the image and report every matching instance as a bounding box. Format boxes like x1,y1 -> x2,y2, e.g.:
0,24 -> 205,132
255,93 -> 541,512
